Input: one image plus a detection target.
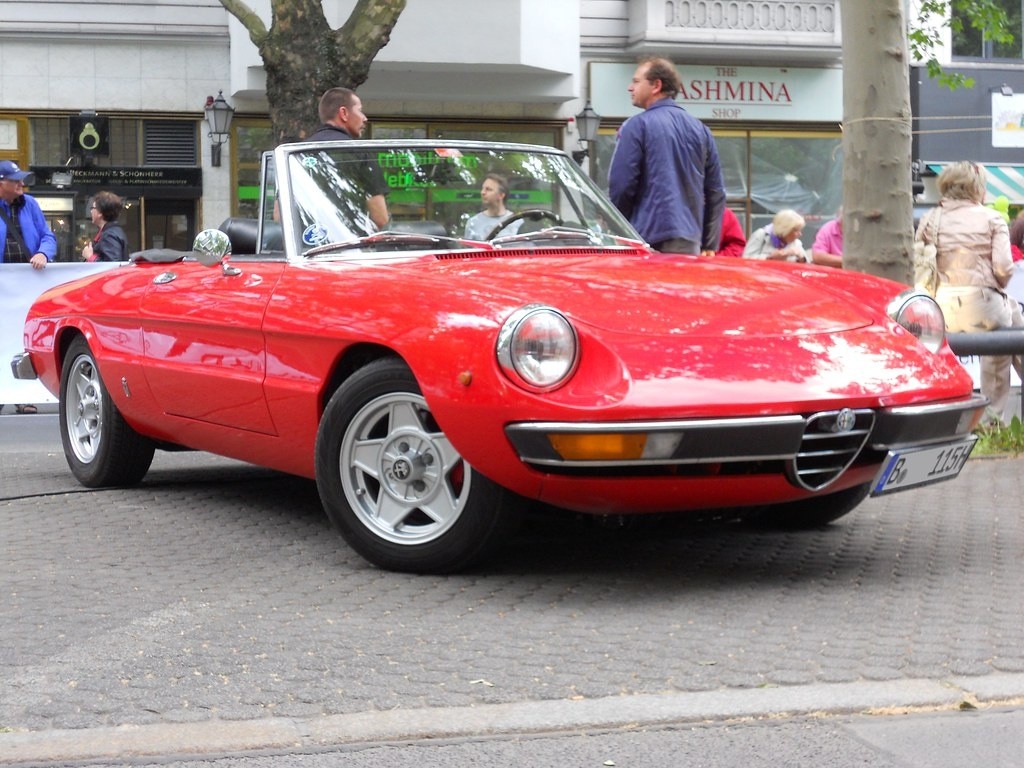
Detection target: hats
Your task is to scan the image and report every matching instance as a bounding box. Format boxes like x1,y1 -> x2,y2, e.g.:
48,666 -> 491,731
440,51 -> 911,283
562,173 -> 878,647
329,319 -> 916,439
0,159 -> 36,179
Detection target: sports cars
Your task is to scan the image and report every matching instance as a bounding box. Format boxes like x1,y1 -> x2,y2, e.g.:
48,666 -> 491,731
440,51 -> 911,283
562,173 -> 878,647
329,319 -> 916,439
11,140 -> 990,575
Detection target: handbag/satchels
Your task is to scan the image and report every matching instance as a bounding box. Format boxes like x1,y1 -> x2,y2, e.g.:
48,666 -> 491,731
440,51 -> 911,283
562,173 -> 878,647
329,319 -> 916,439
913,206 -> 943,299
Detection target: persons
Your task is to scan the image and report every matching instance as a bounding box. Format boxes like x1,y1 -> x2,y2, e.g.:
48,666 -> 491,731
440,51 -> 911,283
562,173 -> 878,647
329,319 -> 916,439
0,161 -> 58,270
912,160 -> 1024,429
606,57 -> 746,258
463,173 -> 526,241
811,218 -> 844,268
82,191 -> 130,262
273,87 -> 392,237
742,210 -> 811,264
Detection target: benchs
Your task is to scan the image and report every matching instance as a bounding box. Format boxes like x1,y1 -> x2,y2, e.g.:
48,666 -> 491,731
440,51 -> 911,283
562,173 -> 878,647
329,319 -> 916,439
948,330 -> 1024,422
217,216 -> 447,256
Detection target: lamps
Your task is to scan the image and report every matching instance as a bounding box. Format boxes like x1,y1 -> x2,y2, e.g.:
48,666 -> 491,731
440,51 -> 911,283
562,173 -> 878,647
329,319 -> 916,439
205,88 -> 237,167
988,83 -> 1014,97
572,98 -> 603,167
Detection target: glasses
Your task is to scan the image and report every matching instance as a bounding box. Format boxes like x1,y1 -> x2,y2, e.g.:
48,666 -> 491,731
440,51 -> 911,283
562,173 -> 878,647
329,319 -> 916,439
90,206 -> 97,209
